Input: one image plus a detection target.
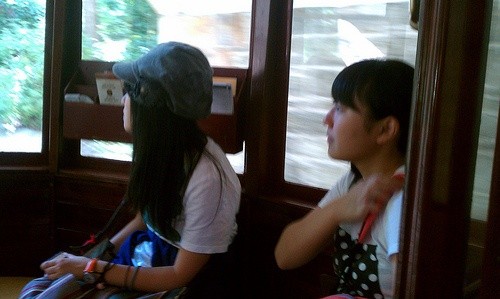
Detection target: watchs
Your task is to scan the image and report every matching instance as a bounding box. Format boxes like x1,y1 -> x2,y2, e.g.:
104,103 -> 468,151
83,258 -> 97,285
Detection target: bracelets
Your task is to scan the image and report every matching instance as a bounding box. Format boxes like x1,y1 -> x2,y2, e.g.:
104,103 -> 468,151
93,261 -> 141,290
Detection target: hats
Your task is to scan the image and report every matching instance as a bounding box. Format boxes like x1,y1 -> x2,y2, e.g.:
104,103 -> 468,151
113,43 -> 213,120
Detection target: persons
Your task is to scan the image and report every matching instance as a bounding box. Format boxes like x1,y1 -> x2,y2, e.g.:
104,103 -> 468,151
274,58 -> 415,299
17,41 -> 242,299
